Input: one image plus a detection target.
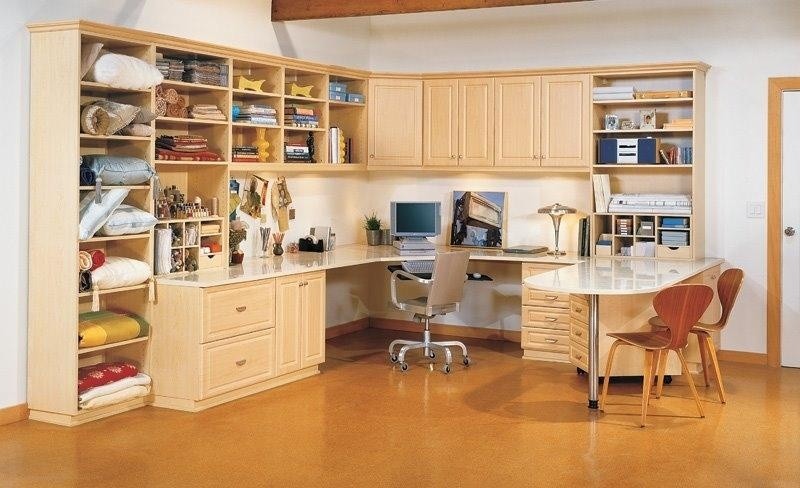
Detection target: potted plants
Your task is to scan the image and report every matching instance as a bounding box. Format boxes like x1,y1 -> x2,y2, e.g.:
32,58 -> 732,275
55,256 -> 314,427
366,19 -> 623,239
364,211 -> 382,245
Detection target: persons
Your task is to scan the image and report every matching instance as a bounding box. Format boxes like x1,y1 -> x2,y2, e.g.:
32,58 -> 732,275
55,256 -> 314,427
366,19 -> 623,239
642,114 -> 653,130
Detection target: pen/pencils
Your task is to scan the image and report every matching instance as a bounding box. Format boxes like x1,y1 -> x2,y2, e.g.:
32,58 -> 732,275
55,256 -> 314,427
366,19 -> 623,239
271,232 -> 286,244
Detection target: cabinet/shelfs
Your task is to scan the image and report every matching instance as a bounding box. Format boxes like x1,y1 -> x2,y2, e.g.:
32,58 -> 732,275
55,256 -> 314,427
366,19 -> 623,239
368,71 -> 423,171
590,61 -> 712,261
495,67 -> 590,173
423,71 -> 495,171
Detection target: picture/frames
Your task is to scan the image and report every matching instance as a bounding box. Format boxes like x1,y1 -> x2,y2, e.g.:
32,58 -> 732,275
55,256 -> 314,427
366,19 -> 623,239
446,188 -> 507,251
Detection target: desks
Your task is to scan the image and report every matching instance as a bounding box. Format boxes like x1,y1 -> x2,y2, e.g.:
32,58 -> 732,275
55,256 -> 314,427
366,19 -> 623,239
524,258 -> 726,408
156,245 -> 589,413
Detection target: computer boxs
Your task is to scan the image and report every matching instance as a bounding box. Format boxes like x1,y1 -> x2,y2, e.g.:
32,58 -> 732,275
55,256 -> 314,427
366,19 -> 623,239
393,241 -> 436,250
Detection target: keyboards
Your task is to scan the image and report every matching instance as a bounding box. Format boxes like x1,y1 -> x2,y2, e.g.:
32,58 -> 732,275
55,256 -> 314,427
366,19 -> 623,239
401,260 -> 435,275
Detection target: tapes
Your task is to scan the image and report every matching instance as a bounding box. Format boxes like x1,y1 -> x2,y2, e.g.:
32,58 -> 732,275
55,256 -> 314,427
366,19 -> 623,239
304,235 -> 319,244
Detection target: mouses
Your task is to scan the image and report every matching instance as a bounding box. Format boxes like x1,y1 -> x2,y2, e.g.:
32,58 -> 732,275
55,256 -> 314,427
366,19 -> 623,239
473,272 -> 482,278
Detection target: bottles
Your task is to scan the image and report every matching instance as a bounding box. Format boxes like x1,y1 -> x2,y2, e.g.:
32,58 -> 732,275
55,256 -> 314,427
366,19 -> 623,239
330,233 -> 336,251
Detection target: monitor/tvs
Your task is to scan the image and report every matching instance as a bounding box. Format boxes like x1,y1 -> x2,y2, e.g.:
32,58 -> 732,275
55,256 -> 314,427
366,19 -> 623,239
390,201 -> 441,244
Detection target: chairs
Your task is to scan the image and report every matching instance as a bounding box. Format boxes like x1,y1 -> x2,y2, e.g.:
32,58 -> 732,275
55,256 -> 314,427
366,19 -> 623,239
646,268 -> 744,406
599,284 -> 714,428
389,250 -> 471,374
